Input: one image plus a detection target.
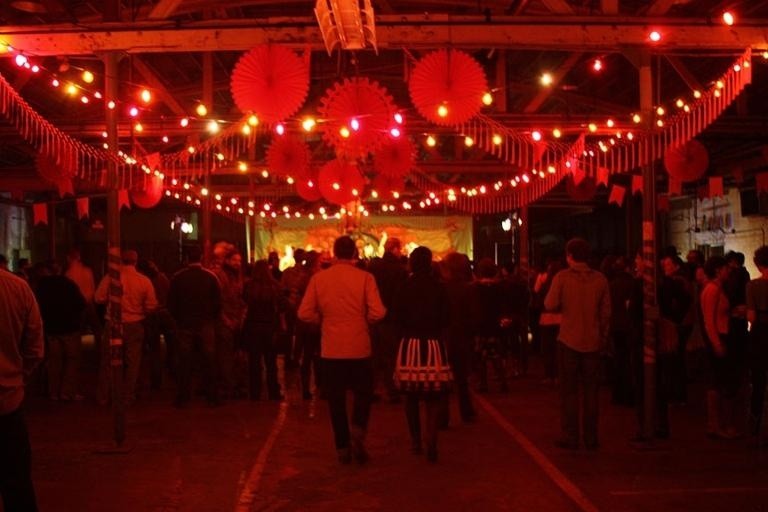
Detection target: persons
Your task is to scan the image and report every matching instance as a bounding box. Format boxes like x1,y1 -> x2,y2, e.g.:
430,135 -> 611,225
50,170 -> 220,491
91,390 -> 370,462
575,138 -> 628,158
0,214 -> 768,512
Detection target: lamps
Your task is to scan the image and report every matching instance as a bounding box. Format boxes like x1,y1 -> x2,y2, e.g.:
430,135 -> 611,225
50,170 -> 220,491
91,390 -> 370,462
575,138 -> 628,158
311,1 -> 383,59
